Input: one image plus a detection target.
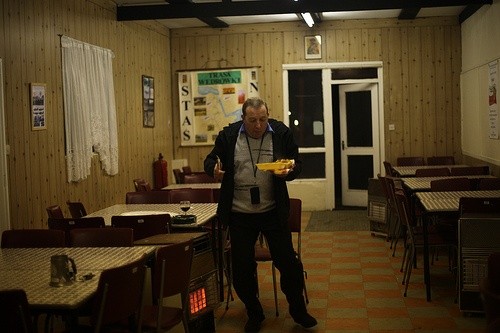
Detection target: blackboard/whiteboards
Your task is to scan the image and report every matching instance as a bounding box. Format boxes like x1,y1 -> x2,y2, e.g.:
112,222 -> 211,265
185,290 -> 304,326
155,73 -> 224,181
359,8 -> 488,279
458,58 -> 500,173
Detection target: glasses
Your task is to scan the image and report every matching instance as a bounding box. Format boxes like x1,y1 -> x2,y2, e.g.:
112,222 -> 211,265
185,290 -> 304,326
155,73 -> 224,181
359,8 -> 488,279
244,117 -> 267,128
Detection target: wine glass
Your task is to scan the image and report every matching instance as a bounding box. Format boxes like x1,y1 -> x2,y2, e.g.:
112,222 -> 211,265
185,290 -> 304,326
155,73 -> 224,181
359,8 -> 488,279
180,201 -> 190,216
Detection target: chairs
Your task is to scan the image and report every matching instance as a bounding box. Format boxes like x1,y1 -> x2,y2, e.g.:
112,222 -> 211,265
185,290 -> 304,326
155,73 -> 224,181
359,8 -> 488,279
378,155 -> 500,308
0,166 -> 309,333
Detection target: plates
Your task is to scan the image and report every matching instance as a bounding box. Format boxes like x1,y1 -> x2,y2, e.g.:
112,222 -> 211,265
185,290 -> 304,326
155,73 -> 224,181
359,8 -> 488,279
256,162 -> 287,171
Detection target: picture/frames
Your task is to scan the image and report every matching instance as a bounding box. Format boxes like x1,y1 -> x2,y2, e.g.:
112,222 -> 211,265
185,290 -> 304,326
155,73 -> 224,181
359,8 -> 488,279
304,35 -> 322,59
30,83 -> 47,130
141,74 -> 156,128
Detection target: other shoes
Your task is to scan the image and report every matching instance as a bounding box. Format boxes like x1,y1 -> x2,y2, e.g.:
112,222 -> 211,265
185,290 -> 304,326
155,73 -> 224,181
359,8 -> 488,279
289,310 -> 318,328
244,315 -> 265,333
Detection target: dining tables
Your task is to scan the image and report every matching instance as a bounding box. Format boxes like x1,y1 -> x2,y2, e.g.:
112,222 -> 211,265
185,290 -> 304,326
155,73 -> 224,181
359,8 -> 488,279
161,183 -> 222,190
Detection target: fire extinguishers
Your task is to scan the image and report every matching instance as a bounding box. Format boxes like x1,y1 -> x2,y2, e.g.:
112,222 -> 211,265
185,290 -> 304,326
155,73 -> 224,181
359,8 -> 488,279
151,154 -> 168,189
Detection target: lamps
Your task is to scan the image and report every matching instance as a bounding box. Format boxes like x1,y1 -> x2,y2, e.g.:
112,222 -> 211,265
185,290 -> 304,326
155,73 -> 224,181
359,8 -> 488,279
297,12 -> 320,28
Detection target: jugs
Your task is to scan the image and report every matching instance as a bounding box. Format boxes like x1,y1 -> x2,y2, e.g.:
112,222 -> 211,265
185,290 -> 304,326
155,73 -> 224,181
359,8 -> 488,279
48,255 -> 77,287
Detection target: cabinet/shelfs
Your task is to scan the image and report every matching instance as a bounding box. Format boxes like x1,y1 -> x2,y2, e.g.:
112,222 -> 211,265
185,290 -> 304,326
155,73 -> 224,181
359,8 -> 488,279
454,210 -> 500,314
368,178 -> 391,238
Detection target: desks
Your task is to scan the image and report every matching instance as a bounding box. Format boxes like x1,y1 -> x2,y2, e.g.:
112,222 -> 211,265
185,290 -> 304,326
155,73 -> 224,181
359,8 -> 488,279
414,190 -> 500,301
391,162 -> 472,176
401,174 -> 494,267
81,201 -> 225,303
0,241 -> 165,332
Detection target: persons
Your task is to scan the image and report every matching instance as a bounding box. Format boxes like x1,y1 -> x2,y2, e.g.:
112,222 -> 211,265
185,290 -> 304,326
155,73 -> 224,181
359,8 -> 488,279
204,97 -> 318,333
